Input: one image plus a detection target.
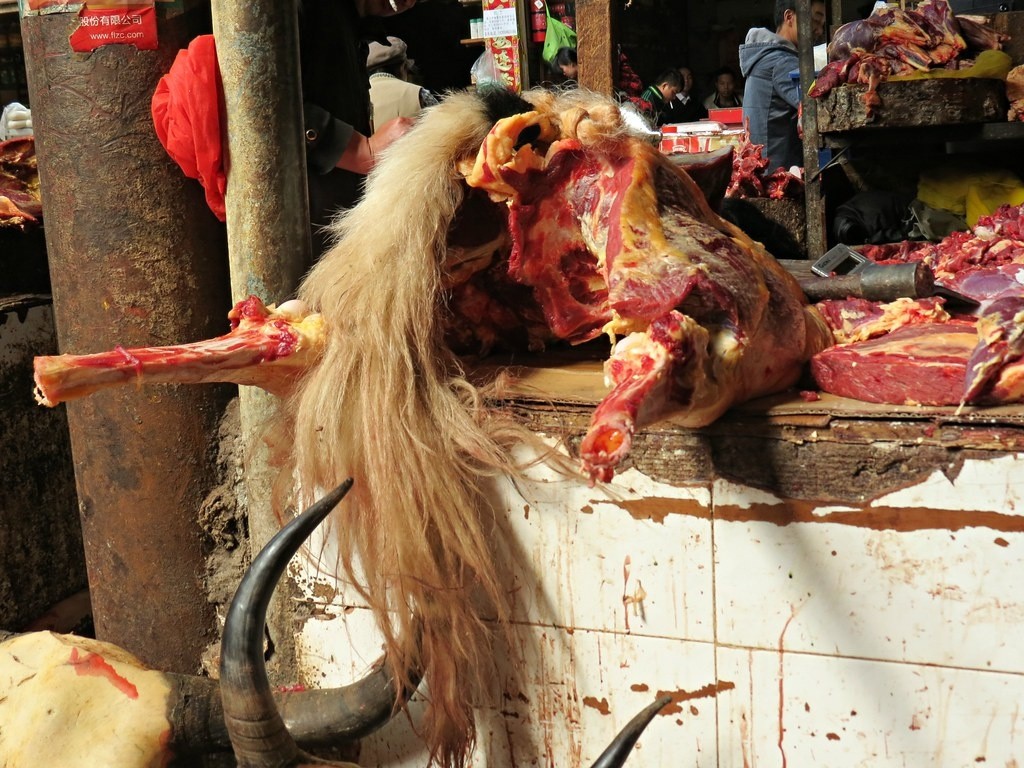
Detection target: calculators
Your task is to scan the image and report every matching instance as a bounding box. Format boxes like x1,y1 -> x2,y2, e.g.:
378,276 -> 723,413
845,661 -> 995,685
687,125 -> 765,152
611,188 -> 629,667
810,243 -> 875,277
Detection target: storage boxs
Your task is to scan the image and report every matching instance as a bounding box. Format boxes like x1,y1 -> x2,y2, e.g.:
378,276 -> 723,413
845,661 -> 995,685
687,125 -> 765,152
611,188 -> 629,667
708,106 -> 744,124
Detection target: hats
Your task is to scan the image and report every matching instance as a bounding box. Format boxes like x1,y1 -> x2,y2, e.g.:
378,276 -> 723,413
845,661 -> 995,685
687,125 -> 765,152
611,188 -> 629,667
366,36 -> 415,71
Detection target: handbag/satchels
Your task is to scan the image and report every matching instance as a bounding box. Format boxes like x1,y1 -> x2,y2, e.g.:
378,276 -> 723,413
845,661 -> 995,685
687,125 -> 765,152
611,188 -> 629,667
543,0 -> 578,66
471,48 -> 502,89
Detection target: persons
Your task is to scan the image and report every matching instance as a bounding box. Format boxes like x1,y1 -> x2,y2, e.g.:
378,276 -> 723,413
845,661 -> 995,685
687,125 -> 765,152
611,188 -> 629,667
557,47 -> 579,86
704,67 -> 743,108
366,36 -> 440,136
663,65 -> 708,124
740,0 -> 826,177
640,68 -> 684,126
303,104 -> 414,176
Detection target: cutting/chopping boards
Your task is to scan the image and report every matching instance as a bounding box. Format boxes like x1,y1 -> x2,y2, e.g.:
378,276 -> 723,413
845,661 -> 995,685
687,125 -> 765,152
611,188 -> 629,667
814,75 -> 1011,132
